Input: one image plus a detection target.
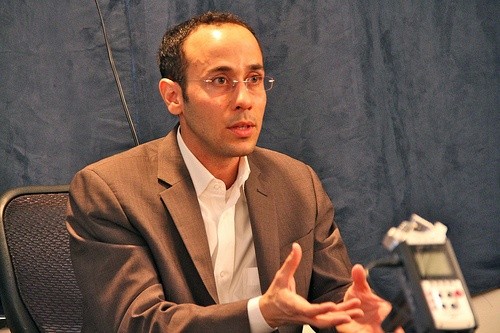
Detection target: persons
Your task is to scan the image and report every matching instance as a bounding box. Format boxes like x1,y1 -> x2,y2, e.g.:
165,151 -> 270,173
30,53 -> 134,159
396,289 -> 500,333
66,10 -> 406,333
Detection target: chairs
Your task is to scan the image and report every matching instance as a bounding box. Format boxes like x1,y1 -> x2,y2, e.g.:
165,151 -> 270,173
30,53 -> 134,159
0,184 -> 85,333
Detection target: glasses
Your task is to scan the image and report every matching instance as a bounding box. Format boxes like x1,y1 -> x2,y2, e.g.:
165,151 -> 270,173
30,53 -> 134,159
176,71 -> 275,94
